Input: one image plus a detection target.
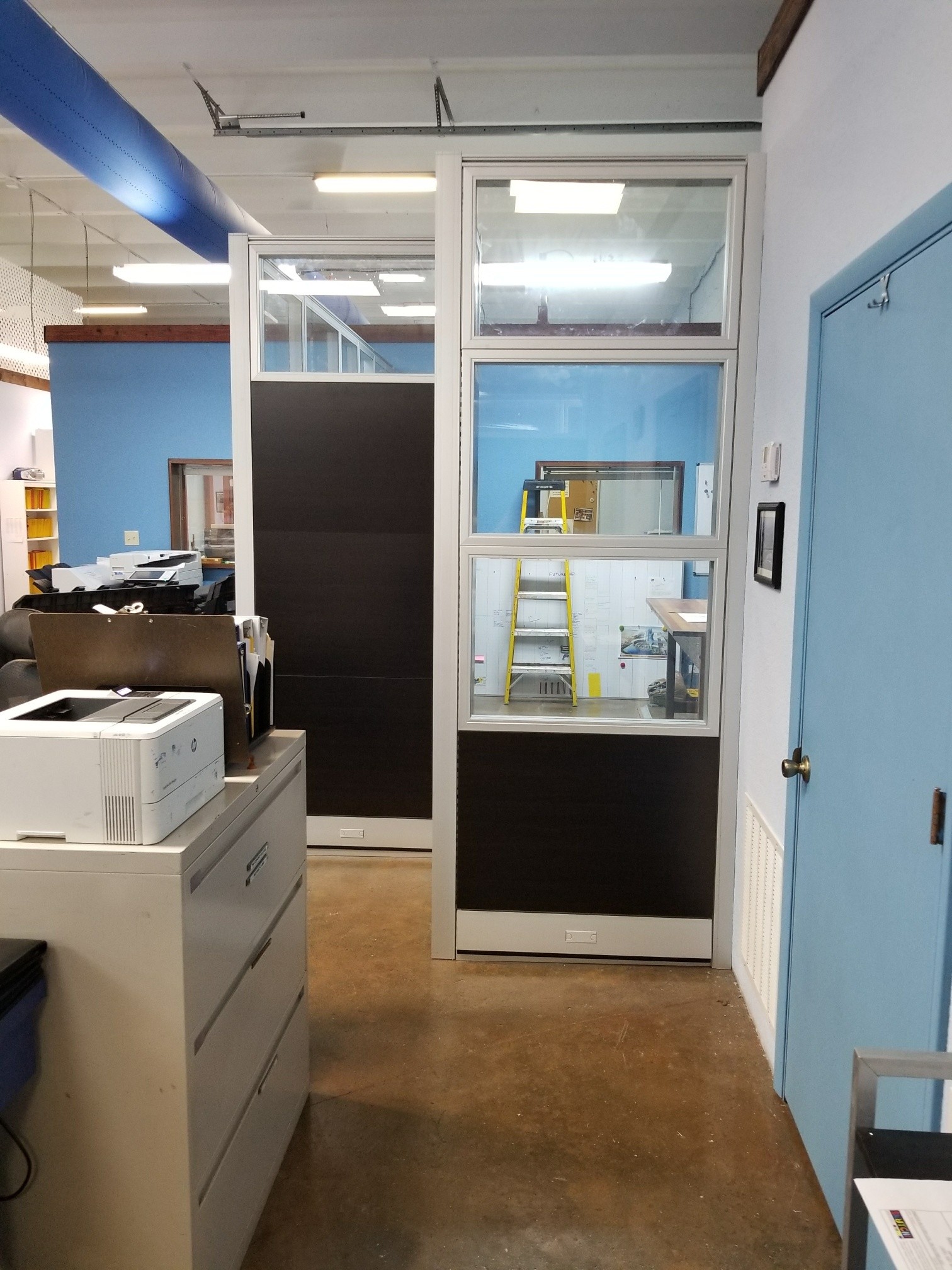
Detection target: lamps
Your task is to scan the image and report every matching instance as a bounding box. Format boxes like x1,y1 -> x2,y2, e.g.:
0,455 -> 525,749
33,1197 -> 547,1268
313,171 -> 437,193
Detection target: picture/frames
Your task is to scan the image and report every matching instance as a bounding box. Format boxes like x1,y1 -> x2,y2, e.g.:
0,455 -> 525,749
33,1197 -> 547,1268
753,502 -> 784,589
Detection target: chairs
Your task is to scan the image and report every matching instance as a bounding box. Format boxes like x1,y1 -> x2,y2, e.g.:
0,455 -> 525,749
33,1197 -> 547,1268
24,562 -> 235,616
0,608 -> 44,713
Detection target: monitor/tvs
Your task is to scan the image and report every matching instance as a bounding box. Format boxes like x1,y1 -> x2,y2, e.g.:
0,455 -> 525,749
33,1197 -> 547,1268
126,570 -> 176,582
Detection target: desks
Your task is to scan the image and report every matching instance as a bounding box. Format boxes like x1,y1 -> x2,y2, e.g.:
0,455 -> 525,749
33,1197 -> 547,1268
646,597 -> 708,719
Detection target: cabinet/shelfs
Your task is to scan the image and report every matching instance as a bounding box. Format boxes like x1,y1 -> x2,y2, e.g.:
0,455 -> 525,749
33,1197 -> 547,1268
0,429 -> 60,612
0,730 -> 313,1270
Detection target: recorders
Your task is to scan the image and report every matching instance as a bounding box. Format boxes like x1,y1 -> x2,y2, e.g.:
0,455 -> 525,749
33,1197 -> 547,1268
12,467 -> 45,481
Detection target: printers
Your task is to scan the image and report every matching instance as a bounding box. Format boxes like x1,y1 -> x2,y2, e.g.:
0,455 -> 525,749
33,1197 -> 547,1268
25,550 -> 203,595
0,688 -> 225,846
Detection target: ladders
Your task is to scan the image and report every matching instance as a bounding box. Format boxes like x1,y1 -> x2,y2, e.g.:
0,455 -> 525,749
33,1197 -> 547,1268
505,479 -> 579,707
